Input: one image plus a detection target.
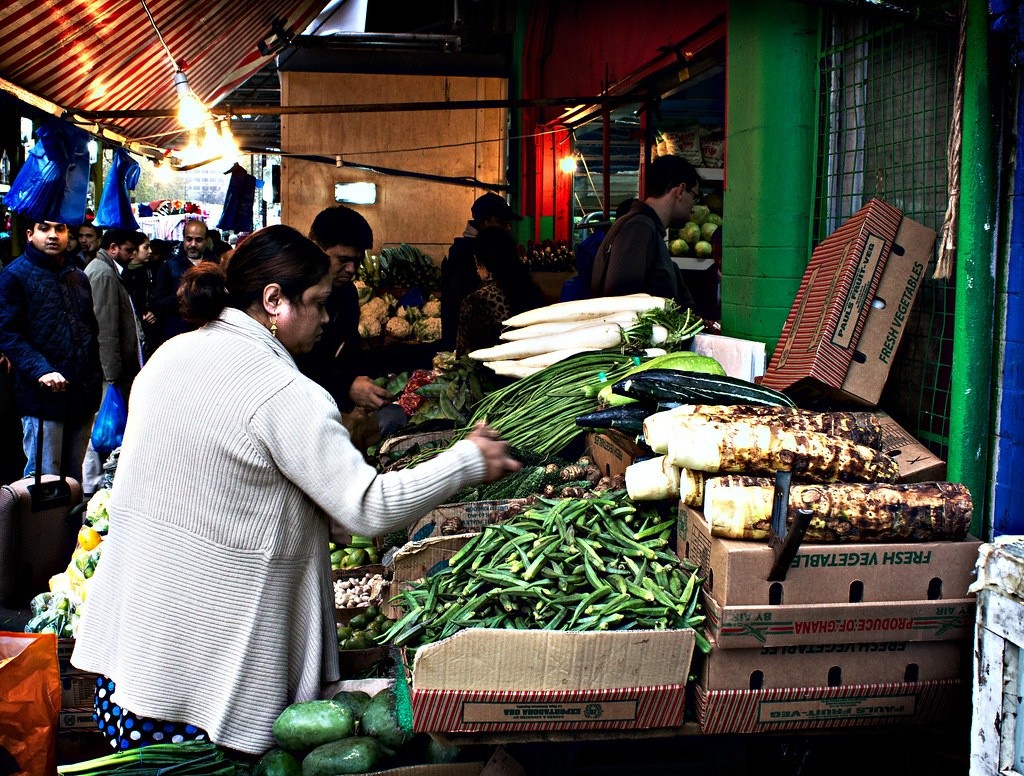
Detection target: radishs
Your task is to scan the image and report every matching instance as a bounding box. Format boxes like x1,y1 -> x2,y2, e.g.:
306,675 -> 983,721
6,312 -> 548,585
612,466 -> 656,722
469,290 -> 700,381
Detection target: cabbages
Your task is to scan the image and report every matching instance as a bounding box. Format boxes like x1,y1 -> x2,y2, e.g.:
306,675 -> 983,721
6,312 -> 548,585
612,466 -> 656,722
669,206 -> 724,257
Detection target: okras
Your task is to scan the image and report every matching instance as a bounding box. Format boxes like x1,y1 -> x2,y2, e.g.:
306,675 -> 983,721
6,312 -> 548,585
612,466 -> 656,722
371,484 -> 715,657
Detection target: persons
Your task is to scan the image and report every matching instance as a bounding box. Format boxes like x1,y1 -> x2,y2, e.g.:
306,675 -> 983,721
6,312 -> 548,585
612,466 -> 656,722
455,227 -> 545,361
71,224 -> 522,763
156,220 -> 221,342
0,211 -> 104,479
63,221 -> 180,296
695,225 -> 721,323
293,204 -> 397,415
576,198 -> 642,299
122,232 -> 161,355
83,226 -> 146,497
440,192 -> 524,352
590,154 -> 701,313
206,230 -> 233,258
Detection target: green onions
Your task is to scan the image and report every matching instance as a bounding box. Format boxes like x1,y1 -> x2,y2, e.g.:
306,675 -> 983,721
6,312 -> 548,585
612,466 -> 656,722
56,741 -> 252,776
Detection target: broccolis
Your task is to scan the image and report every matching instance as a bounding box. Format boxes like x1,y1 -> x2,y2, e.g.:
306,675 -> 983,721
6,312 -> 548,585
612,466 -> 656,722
351,280 -> 443,341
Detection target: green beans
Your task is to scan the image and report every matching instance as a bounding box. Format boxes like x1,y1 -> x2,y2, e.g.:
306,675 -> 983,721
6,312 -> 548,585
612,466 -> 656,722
398,354 -> 665,457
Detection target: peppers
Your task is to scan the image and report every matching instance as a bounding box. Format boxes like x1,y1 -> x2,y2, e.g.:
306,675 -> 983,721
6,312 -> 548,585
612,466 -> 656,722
378,242 -> 443,285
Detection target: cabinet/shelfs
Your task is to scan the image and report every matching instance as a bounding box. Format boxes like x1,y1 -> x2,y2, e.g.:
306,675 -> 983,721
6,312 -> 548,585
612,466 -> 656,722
670,167 -> 724,272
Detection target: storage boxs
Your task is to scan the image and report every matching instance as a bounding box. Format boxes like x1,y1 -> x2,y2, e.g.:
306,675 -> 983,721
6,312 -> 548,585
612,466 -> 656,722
389,531 -> 697,734
331,563 -> 388,679
839,404 -> 947,482
674,498 -> 986,646
760,197 -> 938,413
690,625 -> 972,737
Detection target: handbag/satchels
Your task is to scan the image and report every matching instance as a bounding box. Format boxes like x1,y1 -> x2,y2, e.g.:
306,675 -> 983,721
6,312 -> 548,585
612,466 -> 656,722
0,630 -> 62,776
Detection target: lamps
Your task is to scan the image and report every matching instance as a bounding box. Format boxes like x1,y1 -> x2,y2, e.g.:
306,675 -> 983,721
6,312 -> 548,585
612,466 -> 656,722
139,0 -> 213,129
219,120 -> 234,138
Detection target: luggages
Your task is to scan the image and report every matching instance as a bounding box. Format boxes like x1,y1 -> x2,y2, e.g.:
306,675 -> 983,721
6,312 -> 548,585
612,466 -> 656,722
1,380 -> 83,610
